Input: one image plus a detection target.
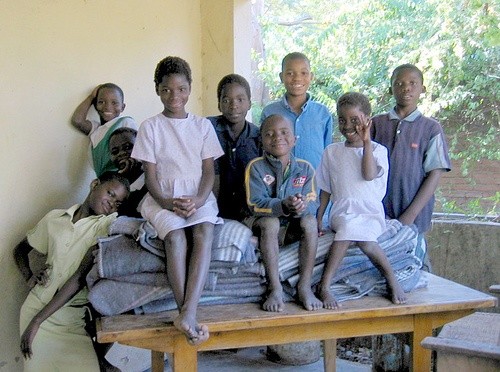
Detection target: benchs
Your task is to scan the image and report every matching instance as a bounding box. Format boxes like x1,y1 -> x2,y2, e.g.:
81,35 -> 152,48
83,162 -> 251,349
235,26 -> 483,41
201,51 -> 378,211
420,312 -> 500,372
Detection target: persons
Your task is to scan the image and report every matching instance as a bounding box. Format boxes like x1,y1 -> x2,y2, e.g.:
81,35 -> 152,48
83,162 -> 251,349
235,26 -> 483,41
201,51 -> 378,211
245,114 -> 324,311
13,171 -> 131,372
108,127 -> 149,218
207,74 -> 262,223
130,56 -> 225,346
260,52 -> 333,174
71,83 -> 140,178
369,63 -> 451,265
317,92 -> 408,309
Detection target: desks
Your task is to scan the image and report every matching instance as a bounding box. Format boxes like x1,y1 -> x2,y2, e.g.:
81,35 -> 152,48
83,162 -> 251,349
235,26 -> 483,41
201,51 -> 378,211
96,269 -> 500,372
